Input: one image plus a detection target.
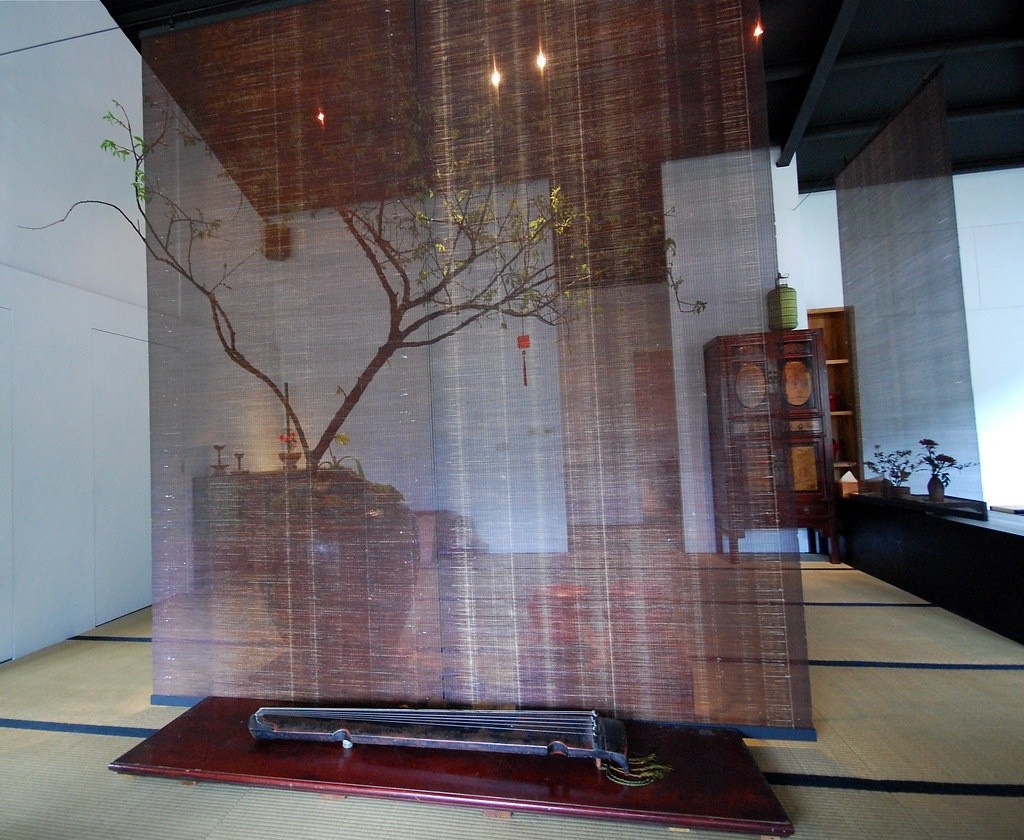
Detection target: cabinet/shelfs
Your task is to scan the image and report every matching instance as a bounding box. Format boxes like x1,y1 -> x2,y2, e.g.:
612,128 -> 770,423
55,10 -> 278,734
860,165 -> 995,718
191,470 -> 313,589
704,304 -> 864,565
843,494 -> 1024,644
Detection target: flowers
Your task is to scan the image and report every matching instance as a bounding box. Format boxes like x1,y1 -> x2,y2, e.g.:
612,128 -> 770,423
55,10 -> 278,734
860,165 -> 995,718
278,427 -> 298,454
919,439 -> 979,487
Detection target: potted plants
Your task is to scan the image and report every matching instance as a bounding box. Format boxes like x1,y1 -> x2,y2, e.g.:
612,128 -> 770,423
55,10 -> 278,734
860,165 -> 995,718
862,443 -> 920,496
14,85 -> 705,679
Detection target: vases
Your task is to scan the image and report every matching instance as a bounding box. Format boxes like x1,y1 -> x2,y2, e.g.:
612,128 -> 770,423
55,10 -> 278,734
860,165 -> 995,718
927,473 -> 944,502
278,452 -> 301,470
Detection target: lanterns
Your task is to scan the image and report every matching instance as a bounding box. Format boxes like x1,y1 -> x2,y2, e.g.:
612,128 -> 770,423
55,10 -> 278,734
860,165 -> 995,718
766,273 -> 799,332
